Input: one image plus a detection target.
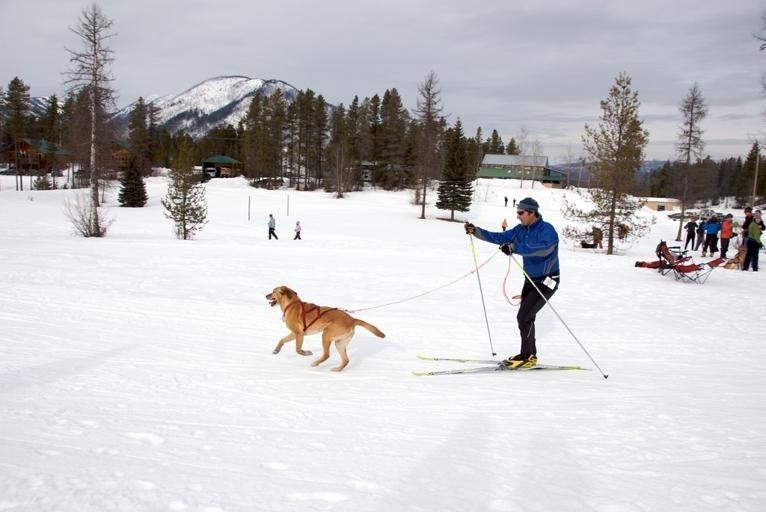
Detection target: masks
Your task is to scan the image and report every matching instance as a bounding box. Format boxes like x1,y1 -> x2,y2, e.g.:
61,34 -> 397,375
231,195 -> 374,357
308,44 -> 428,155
754,218 -> 761,224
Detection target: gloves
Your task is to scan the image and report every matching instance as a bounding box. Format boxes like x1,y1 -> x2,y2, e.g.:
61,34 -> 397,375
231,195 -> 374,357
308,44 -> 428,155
499,240 -> 515,255
464,223 -> 476,234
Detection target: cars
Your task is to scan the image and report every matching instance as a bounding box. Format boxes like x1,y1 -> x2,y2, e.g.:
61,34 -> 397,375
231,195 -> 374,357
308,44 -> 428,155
203,166 -> 216,179
669,207 -> 727,225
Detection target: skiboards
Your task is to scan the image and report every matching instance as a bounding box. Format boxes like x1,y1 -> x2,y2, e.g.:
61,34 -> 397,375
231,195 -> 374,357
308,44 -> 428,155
411,356 -> 593,376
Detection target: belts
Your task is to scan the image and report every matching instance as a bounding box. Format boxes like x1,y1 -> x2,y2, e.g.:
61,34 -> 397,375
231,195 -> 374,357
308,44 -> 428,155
552,274 -> 560,279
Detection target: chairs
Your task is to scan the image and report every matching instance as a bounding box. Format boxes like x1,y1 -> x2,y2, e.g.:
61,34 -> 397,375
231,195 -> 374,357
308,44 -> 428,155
655,239 -> 726,285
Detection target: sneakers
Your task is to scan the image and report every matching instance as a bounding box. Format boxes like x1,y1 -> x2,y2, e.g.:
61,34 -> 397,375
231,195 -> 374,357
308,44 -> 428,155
509,355 -> 537,366
503,360 -> 533,369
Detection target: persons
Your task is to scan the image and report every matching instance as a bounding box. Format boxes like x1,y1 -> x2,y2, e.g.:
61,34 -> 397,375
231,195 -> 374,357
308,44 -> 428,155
513,198 -> 517,207
681,205 -> 766,273
267,213 -> 280,241
502,219 -> 508,231
585,225 -> 604,249
504,196 -> 509,207
462,195 -> 562,368
291,221 -> 302,240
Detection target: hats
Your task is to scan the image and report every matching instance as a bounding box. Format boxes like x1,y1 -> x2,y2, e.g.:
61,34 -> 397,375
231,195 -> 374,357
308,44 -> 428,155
751,213 -> 761,219
516,197 -> 538,211
745,206 -> 752,213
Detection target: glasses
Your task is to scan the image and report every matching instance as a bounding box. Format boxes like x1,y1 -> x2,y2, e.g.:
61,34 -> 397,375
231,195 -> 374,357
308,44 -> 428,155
517,209 -> 533,215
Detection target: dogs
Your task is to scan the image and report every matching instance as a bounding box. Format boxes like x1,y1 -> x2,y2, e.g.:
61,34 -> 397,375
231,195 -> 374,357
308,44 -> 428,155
724,245 -> 746,269
266,286 -> 385,372
